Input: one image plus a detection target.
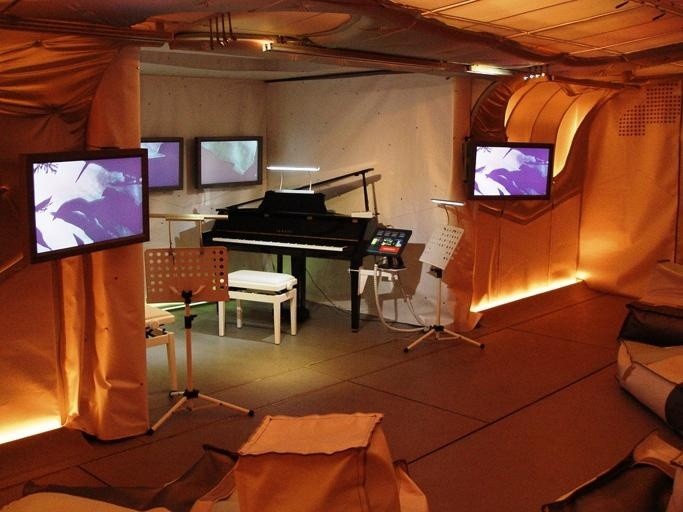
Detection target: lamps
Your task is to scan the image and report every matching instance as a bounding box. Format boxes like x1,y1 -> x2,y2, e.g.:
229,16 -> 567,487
210,12 -> 238,51
523,65 -> 545,81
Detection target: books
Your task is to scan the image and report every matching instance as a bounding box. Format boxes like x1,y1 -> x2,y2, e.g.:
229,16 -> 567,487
350,211 -> 373,218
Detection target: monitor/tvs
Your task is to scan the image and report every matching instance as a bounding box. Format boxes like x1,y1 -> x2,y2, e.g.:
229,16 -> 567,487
466,140 -> 555,200
365,228 -> 413,269
24,147 -> 150,265
140,137 -> 184,191
195,136 -> 263,190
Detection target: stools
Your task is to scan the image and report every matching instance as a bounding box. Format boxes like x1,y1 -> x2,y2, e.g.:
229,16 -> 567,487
145,303 -> 178,391
218,269 -> 298,345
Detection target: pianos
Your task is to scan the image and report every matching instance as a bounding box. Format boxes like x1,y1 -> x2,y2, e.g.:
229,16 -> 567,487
201,167 -> 378,334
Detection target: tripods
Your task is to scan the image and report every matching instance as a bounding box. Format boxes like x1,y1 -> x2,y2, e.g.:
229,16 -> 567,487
145,284 -> 255,436
403,265 -> 485,354
